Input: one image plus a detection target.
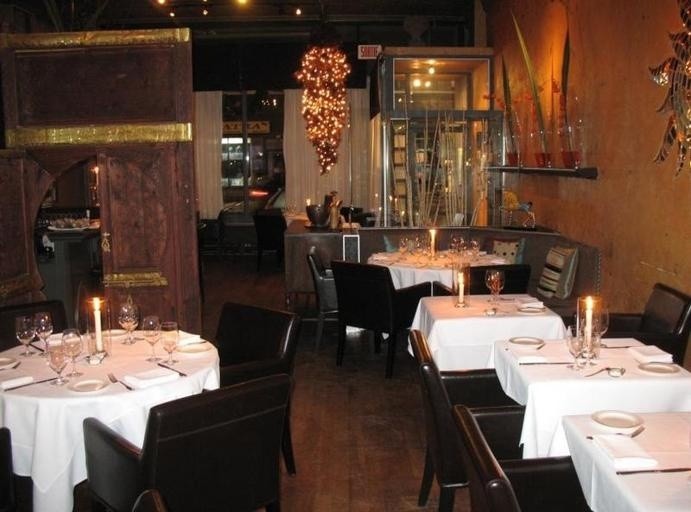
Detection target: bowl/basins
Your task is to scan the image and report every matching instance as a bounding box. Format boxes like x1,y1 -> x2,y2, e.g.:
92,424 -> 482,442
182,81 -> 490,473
306,204 -> 329,225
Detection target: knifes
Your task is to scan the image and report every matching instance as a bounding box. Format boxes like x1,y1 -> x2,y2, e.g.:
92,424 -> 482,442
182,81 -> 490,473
614,467 -> 691,474
4,375 -> 62,392
158,362 -> 187,378
520,361 -> 575,366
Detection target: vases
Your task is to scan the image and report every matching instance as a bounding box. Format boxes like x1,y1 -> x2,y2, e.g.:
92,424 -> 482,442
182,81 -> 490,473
525,96 -> 559,168
557,87 -> 589,168
491,103 -> 521,168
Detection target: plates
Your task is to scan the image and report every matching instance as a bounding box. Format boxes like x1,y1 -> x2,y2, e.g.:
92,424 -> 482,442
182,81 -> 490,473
518,307 -> 546,314
593,410 -> 645,430
66,376 -> 110,393
175,344 -> 213,355
108,373 -> 134,391
0,357 -> 18,370
639,361 -> 682,373
510,336 -> 545,346
98,328 -> 128,339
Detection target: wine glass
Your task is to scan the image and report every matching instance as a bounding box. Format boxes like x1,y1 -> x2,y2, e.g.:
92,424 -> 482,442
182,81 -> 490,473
118,301 -> 179,366
567,325 -> 585,372
46,325 -> 81,386
398,237 -> 481,262
485,269 -> 506,308
16,312 -> 54,358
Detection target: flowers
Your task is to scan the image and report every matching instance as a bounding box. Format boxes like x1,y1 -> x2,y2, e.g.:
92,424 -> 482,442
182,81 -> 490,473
511,13 -> 552,154
550,29 -> 572,152
483,55 -> 519,151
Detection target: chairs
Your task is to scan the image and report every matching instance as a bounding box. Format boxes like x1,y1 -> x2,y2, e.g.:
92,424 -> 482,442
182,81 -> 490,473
250,207 -> 287,273
